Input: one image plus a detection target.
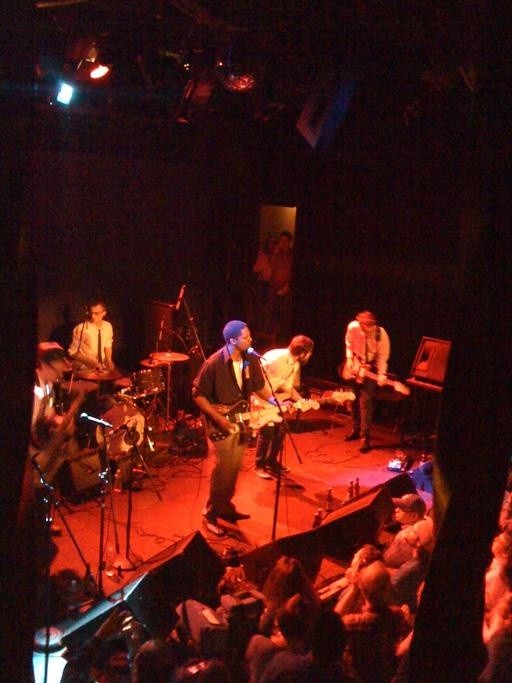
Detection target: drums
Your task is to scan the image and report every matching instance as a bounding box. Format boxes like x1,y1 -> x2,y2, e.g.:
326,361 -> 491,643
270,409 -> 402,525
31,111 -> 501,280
131,368 -> 166,396
89,394 -> 146,459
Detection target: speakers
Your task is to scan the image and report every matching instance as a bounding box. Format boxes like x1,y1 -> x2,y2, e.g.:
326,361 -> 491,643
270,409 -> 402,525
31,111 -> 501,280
340,471 -> 420,523
119,530 -> 225,602
412,459 -> 434,495
410,336 -> 453,385
238,522 -> 338,591
317,501 -> 390,557
61,570 -> 178,657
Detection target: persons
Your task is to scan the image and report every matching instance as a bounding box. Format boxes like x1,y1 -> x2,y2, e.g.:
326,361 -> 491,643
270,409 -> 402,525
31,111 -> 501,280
27,232 -> 511,682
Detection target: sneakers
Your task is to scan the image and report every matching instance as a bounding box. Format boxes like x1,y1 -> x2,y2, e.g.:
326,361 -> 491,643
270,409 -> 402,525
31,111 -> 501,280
201,504 -> 251,538
252,456 -> 291,480
344,426 -> 372,453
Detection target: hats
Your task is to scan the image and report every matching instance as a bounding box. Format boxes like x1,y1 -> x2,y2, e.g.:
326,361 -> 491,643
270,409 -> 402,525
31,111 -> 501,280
392,493 -> 427,517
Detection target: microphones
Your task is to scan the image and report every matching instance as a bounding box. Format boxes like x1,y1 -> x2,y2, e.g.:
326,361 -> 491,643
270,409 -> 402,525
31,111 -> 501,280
80,412 -> 116,428
174,283 -> 188,311
246,347 -> 265,361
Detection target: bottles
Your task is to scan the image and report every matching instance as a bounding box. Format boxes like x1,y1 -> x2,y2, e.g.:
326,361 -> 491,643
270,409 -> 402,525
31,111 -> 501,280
354,477 -> 363,495
325,487 -> 333,511
422,449 -> 426,462
312,509 -> 322,527
346,481 -> 354,498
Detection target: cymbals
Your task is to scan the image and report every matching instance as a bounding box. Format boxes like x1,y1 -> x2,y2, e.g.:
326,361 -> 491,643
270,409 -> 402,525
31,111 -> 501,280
141,358 -> 160,367
61,381 -> 97,392
77,369 -> 120,381
149,352 -> 190,362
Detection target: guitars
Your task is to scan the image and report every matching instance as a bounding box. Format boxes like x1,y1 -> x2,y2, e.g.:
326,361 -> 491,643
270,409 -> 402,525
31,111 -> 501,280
25,396 -> 84,500
201,399 -> 320,441
250,387 -> 356,410
342,357 -> 410,396
